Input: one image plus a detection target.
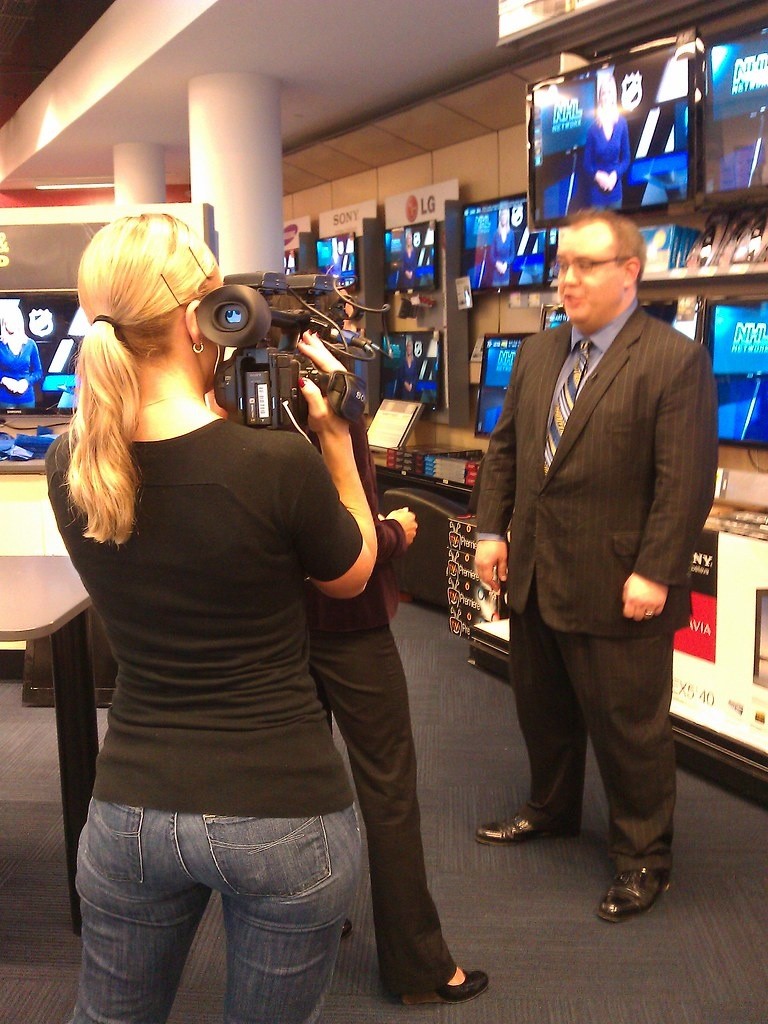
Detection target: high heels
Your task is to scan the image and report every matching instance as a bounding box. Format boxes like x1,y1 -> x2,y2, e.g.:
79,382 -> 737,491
402,968 -> 489,1006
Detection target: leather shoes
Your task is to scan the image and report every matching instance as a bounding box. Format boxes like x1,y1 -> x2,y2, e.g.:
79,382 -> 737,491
598,868 -> 672,922
475,812 -> 563,845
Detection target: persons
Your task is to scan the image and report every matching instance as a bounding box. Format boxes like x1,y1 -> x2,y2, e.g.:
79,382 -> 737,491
329,237 -> 343,275
47,216 -> 377,1023
265,271 -> 490,1006
474,209 -> 719,920
0,307 -> 44,408
399,340 -> 417,402
492,206 -> 514,286
403,230 -> 420,284
581,76 -> 631,208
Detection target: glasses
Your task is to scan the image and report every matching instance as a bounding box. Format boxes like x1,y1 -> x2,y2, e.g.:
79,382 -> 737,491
549,257 -> 623,275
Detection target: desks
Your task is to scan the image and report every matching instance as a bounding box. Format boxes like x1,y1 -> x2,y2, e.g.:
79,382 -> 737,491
0,554 -> 104,936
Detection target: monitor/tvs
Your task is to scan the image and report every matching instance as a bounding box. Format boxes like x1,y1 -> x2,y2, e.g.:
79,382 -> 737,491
290,23 -> 768,446
0,288 -> 92,415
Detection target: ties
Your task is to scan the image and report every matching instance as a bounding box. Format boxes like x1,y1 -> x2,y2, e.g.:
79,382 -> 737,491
544,340 -> 591,476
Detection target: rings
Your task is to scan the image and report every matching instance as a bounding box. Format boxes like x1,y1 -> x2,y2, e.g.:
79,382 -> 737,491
644,609 -> 653,616
